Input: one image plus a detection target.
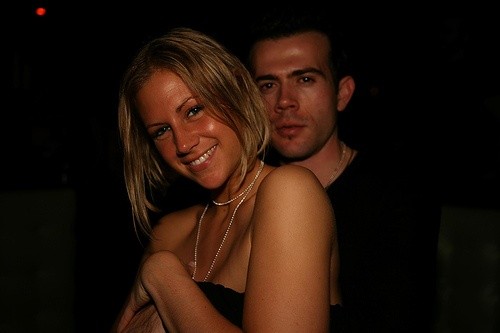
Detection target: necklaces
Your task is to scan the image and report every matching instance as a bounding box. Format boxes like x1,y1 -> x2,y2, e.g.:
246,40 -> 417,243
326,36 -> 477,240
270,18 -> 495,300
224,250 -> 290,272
316,143 -> 354,190
190,161 -> 265,282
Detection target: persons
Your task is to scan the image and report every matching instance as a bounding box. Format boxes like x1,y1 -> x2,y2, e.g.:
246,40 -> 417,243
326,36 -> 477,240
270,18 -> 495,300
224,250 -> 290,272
110,29 -> 342,333
245,19 -> 440,333
